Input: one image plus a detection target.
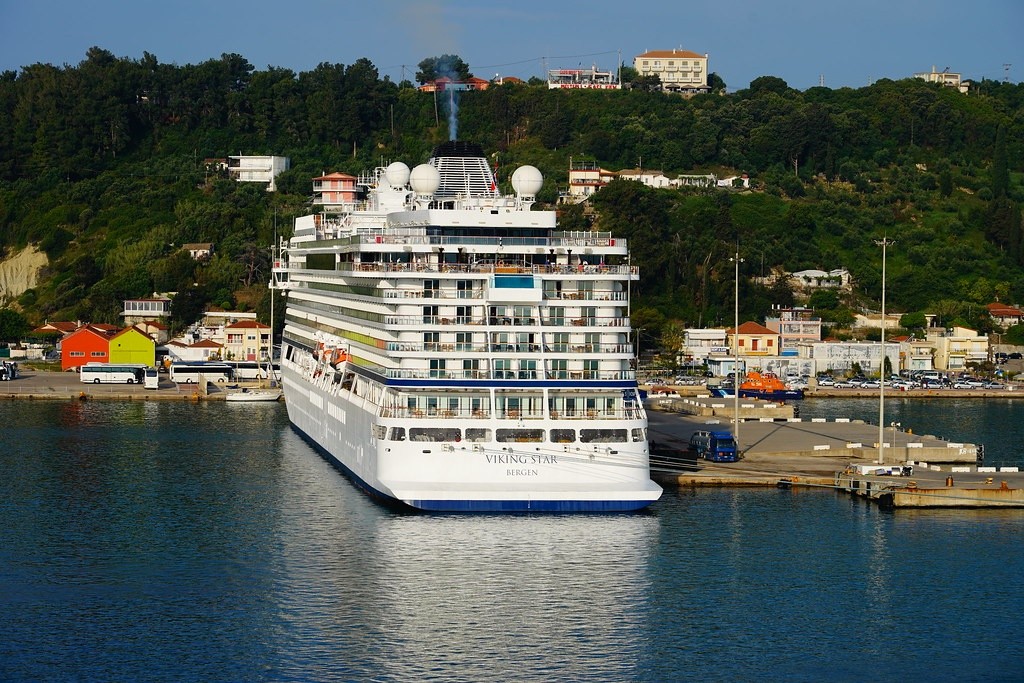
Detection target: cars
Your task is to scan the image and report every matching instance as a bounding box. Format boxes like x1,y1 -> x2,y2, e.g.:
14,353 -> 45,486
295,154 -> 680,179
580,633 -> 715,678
995,352 -> 1008,363
834,379 -> 857,389
636,370 -> 714,387
727,372 -> 736,381
892,381 -> 914,389
816,373 -> 836,386
845,378 -> 864,388
860,380 -> 881,389
782,367 -> 812,388
1007,352 -> 1023,360
875,378 -> 891,386
891,368 -> 1007,389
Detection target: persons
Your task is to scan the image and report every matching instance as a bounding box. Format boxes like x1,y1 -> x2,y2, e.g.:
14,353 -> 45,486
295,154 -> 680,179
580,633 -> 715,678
325,221 -> 338,239
397,259 -> 607,274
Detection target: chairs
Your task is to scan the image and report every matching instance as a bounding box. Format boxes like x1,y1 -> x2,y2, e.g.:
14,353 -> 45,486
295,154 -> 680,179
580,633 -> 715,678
408,292 -> 594,419
360,262 -> 635,274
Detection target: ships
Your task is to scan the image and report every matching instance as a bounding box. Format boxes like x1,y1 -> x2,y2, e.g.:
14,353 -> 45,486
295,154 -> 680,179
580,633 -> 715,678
267,149 -> 665,518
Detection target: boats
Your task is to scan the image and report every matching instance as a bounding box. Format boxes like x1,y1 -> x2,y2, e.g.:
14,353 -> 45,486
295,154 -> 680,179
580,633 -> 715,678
313,339 -> 337,365
706,365 -> 806,404
330,347 -> 353,371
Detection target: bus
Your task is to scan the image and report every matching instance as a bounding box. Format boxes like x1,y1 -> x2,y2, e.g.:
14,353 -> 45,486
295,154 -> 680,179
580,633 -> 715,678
687,428 -> 739,463
80,361 -> 149,385
141,367 -> 159,389
155,354 -> 282,384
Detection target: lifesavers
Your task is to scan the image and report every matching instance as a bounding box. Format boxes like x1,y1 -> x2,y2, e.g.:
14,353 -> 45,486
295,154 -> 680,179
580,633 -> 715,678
324,349 -> 333,360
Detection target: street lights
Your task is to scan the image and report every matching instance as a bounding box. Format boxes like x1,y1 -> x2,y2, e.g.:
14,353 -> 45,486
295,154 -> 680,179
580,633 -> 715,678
630,328 -> 647,377
890,422 -> 901,464
727,253 -> 746,446
873,238 -> 897,465
